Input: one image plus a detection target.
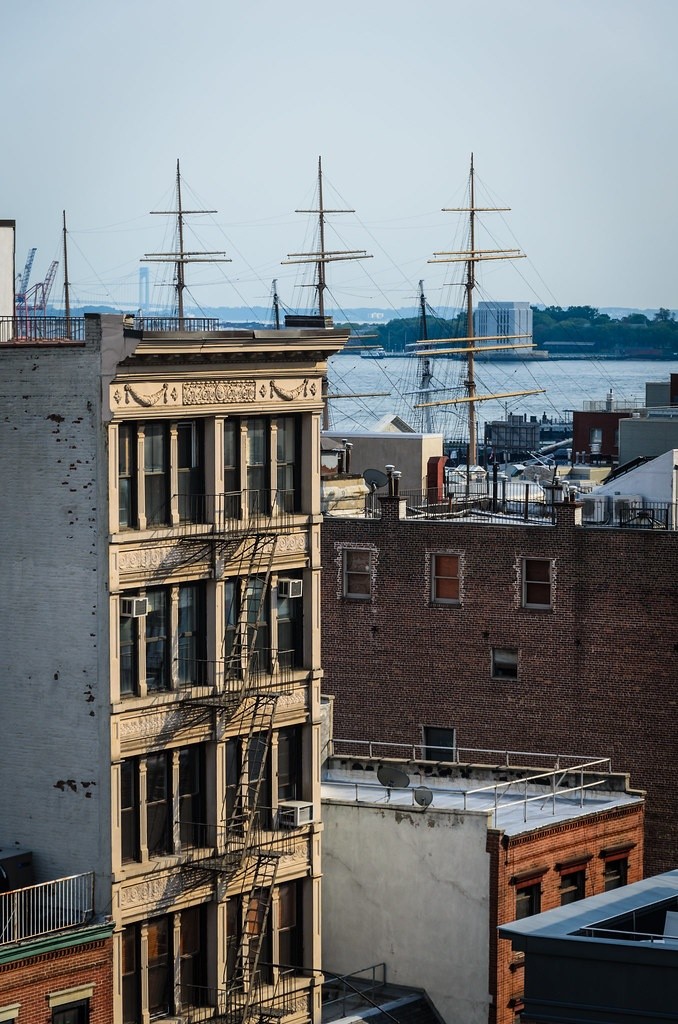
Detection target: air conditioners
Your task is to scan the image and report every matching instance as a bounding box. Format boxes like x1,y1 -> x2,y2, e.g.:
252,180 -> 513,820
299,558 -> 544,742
579,495 -> 609,522
120,597 -> 147,618
279,802 -> 313,826
279,579 -> 302,598
612,494 -> 643,524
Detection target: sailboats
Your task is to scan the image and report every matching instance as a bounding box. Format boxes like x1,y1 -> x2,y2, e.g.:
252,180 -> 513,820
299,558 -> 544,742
402,152 -> 678,530
